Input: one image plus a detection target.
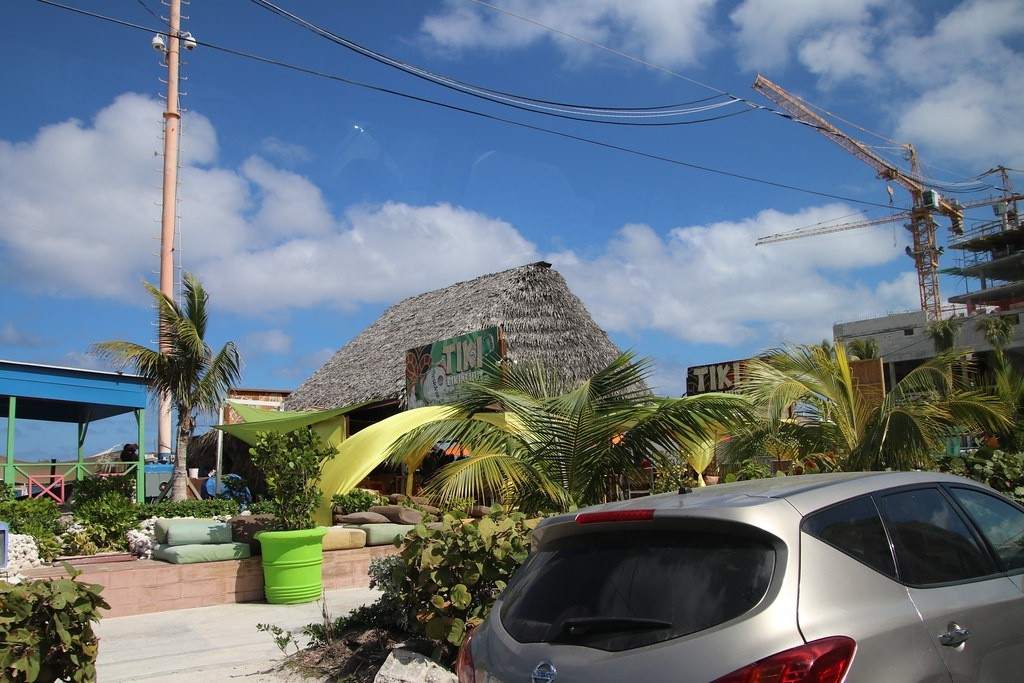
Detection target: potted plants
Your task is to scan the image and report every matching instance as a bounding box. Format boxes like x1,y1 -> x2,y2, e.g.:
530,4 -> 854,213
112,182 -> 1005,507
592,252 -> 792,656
248,426 -> 340,606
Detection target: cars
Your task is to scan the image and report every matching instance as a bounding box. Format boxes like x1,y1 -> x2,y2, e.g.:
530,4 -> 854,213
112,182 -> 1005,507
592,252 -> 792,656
457,471 -> 1024,682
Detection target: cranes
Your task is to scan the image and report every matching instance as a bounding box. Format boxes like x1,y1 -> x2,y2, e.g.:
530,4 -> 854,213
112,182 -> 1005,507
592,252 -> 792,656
755,165 -> 1024,246
752,72 -> 965,321
